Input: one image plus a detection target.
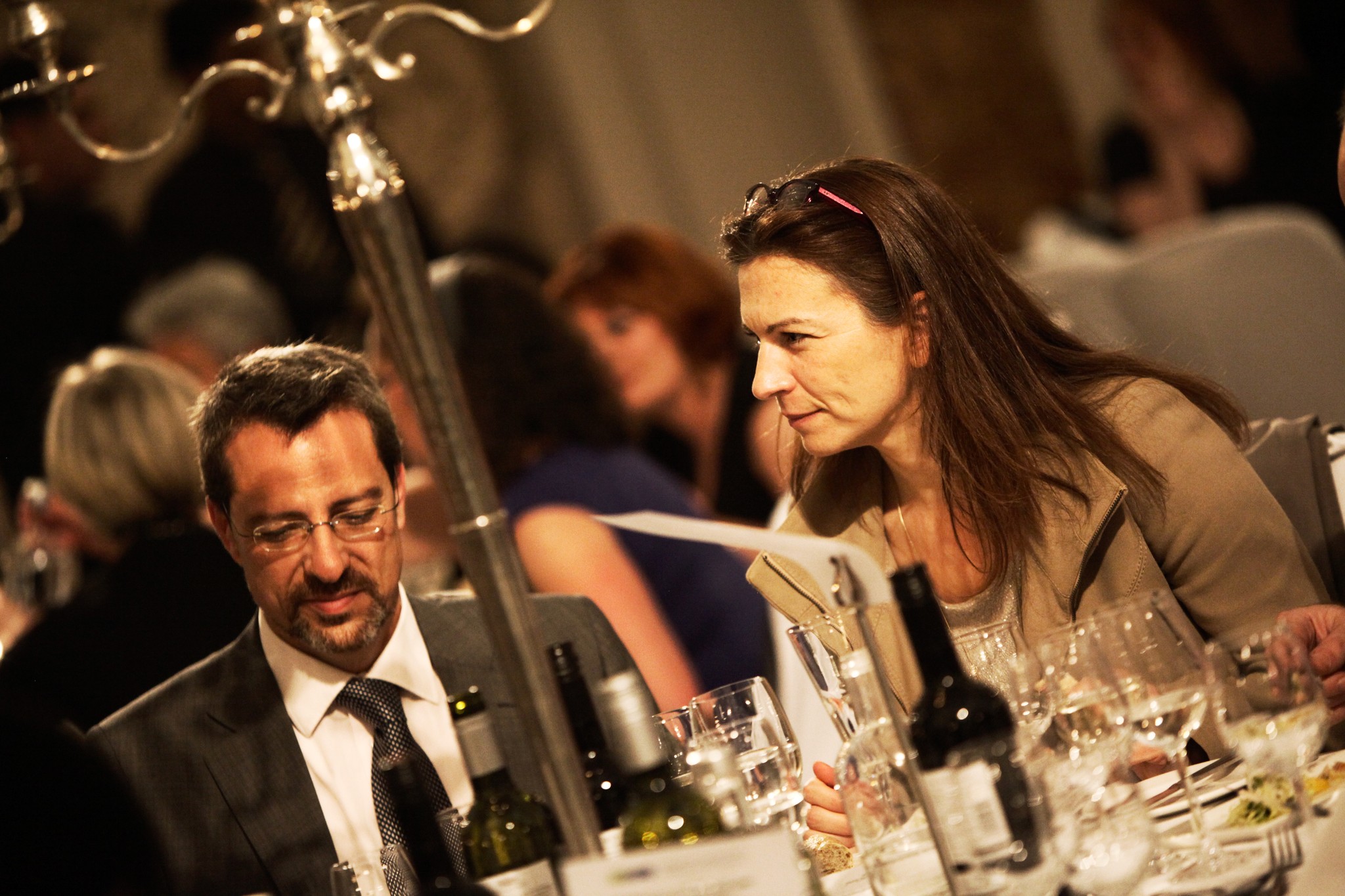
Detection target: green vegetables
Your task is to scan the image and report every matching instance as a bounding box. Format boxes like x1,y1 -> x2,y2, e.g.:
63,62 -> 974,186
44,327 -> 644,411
1238,773 -> 1284,823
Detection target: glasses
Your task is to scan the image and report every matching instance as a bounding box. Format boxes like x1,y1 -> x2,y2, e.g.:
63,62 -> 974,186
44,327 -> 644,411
222,464 -> 400,553
742,184 -> 869,224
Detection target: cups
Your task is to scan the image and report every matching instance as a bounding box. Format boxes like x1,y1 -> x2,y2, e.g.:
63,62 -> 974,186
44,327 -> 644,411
436,802 -> 473,884
0,526 -> 77,617
1040,748 -> 1157,896
649,675 -> 801,822
330,846 -> 419,896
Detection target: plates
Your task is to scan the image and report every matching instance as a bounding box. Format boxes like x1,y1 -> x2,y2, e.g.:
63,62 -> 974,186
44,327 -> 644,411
1195,750 -> 1345,849
1136,759 -> 1266,818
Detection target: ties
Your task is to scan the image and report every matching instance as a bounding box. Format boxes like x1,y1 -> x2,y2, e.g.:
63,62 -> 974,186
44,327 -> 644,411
335,677 -> 474,896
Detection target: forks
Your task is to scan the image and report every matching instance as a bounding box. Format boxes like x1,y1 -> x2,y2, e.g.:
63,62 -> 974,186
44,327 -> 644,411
1225,824 -> 1303,896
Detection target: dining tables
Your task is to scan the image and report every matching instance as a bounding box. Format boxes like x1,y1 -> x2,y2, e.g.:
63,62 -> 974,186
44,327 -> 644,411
813,748 -> 1345,896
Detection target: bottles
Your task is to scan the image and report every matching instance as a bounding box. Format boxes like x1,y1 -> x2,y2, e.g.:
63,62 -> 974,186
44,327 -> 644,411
888,559 -> 1043,883
544,641 -> 729,855
831,647 -> 958,896
446,680 -> 572,895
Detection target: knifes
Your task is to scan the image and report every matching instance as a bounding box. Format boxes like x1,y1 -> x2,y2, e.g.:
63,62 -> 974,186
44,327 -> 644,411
1147,751 -> 1237,805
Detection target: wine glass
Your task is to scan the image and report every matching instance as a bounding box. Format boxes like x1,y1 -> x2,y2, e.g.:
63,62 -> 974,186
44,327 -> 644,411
784,608 -> 901,829
954,587 -> 1331,886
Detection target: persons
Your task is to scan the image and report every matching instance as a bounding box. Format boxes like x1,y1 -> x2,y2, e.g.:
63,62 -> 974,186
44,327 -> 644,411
72,341 -> 684,896
718,154 -> 1345,873
0,0 -> 1345,896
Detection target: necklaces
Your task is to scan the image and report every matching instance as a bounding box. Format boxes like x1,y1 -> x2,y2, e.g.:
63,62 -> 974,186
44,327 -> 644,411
883,467 -> 1000,600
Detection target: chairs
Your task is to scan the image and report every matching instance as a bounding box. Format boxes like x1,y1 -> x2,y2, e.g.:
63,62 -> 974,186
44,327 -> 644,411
1017,216 -> 1345,431
